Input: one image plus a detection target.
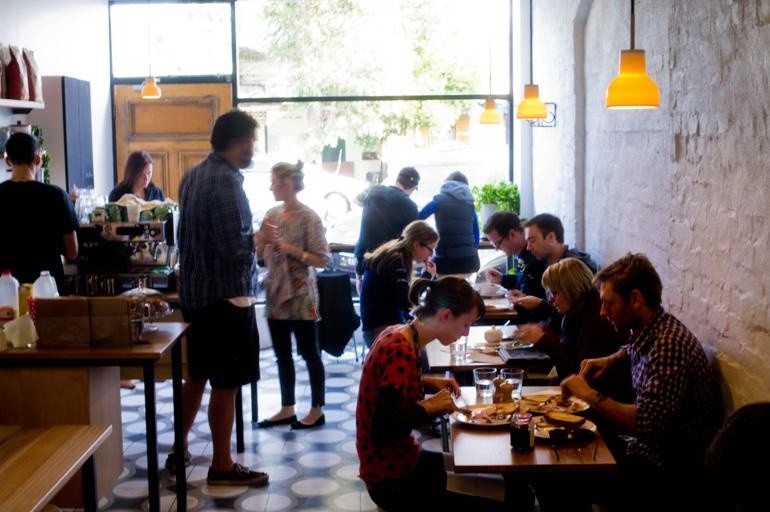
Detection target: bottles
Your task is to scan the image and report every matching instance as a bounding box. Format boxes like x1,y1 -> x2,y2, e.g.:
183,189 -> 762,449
0,270 -> 21,329
18,283 -> 34,315
31,270 -> 60,297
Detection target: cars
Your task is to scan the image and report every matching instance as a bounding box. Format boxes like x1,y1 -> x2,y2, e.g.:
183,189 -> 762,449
237,156 -> 372,219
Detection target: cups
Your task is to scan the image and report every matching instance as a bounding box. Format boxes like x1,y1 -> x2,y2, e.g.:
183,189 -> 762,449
500,368 -> 525,403
126,204 -> 141,223
450,354 -> 473,365
72,186 -> 109,224
473,367 -> 498,399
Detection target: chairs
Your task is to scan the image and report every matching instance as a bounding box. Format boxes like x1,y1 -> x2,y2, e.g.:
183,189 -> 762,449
316,271 -> 367,364
688,344 -> 769,443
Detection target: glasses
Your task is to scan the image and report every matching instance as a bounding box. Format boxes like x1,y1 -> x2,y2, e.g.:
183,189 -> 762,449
489,234 -> 506,248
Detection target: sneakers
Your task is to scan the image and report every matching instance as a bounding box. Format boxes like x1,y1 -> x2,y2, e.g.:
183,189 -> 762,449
206,462 -> 268,487
166,451 -> 193,470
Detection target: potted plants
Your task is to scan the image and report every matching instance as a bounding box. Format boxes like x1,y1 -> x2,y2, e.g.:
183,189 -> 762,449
472,180 -> 522,229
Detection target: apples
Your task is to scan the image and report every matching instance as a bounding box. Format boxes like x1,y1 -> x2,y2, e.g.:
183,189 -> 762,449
141,211 -> 154,221
155,204 -> 168,220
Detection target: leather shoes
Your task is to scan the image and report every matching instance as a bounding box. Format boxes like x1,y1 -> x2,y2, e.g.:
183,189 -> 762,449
290,414 -> 326,429
257,416 -> 297,427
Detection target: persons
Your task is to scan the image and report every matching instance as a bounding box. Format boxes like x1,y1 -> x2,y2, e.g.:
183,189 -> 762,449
107,149 -> 165,390
354,166 -> 420,296
0,132 -> 78,296
258,160 -> 333,429
357,275 -> 536,511
165,107 -> 279,487
358,218 -> 441,373
420,171 -> 481,274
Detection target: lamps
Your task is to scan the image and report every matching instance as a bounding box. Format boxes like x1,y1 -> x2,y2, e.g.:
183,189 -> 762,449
479,69 -> 500,126
137,61 -> 164,100
603,0 -> 664,110
514,1 -> 549,122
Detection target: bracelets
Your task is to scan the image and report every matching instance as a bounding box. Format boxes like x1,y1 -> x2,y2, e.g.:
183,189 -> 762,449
301,251 -> 308,262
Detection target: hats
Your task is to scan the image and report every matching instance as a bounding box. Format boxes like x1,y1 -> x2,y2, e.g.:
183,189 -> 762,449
398,166 -> 421,192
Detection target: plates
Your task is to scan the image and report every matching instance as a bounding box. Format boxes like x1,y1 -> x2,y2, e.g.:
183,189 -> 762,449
471,281 -> 514,311
449,326 -> 535,353
450,392 -> 597,452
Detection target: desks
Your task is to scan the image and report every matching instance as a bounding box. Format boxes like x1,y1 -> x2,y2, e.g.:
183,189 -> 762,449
0,321 -> 191,511
3,417 -> 116,510
153,293 -> 261,454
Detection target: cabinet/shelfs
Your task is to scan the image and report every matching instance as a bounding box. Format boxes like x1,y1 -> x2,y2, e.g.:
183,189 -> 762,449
33,74 -> 96,200
